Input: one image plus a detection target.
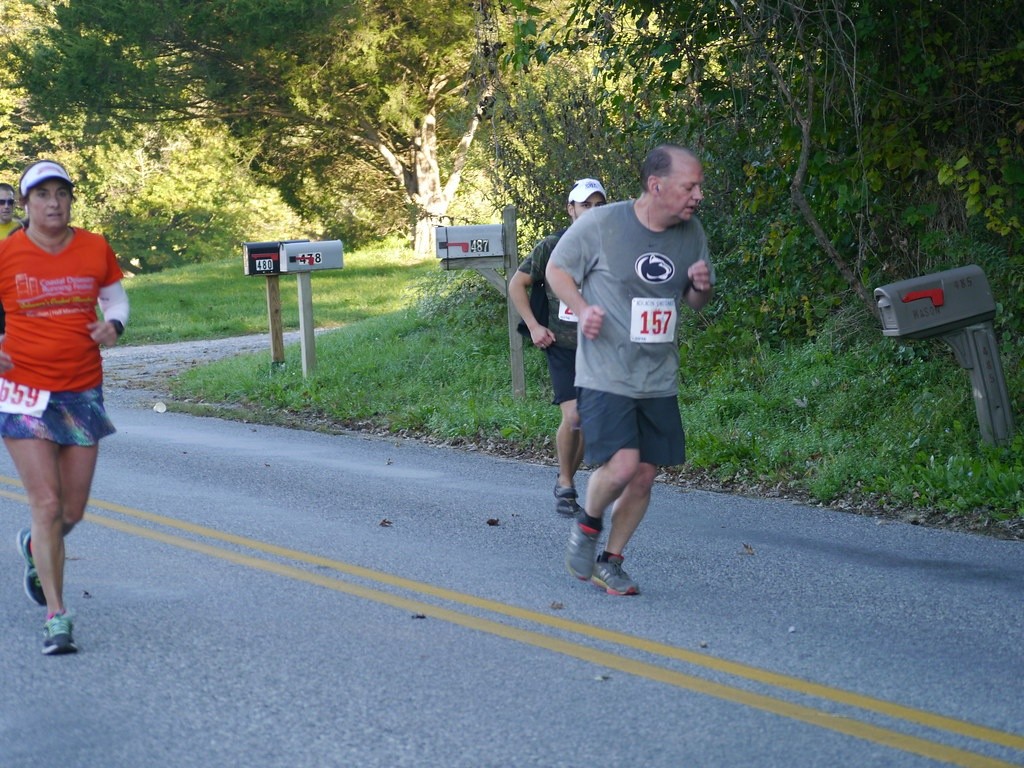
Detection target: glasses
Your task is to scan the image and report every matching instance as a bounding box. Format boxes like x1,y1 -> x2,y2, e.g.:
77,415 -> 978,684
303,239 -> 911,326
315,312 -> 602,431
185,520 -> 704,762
0,199 -> 14,205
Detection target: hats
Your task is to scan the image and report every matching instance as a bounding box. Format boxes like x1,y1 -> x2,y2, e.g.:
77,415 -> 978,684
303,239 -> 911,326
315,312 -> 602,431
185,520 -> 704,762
569,178 -> 606,203
21,162 -> 75,198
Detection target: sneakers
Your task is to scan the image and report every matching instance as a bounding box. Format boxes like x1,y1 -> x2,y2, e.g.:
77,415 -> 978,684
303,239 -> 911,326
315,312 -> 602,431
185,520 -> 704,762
553,489 -> 579,518
17,526 -> 48,607
591,552 -> 640,595
565,511 -> 603,580
553,473 -> 579,497
40,608 -> 77,654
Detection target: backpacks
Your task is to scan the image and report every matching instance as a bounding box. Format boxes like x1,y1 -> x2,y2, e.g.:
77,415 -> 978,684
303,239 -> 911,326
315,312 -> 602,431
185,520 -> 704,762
517,228 -> 564,338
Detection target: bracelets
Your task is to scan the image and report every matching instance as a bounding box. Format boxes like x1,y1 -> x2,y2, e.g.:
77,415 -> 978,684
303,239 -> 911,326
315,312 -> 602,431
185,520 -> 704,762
110,319 -> 125,336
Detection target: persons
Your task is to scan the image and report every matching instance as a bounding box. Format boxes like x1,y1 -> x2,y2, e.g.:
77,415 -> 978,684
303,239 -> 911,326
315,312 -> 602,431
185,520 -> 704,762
543,143 -> 715,598
507,177 -> 609,518
0,159 -> 131,656
0,182 -> 24,337
